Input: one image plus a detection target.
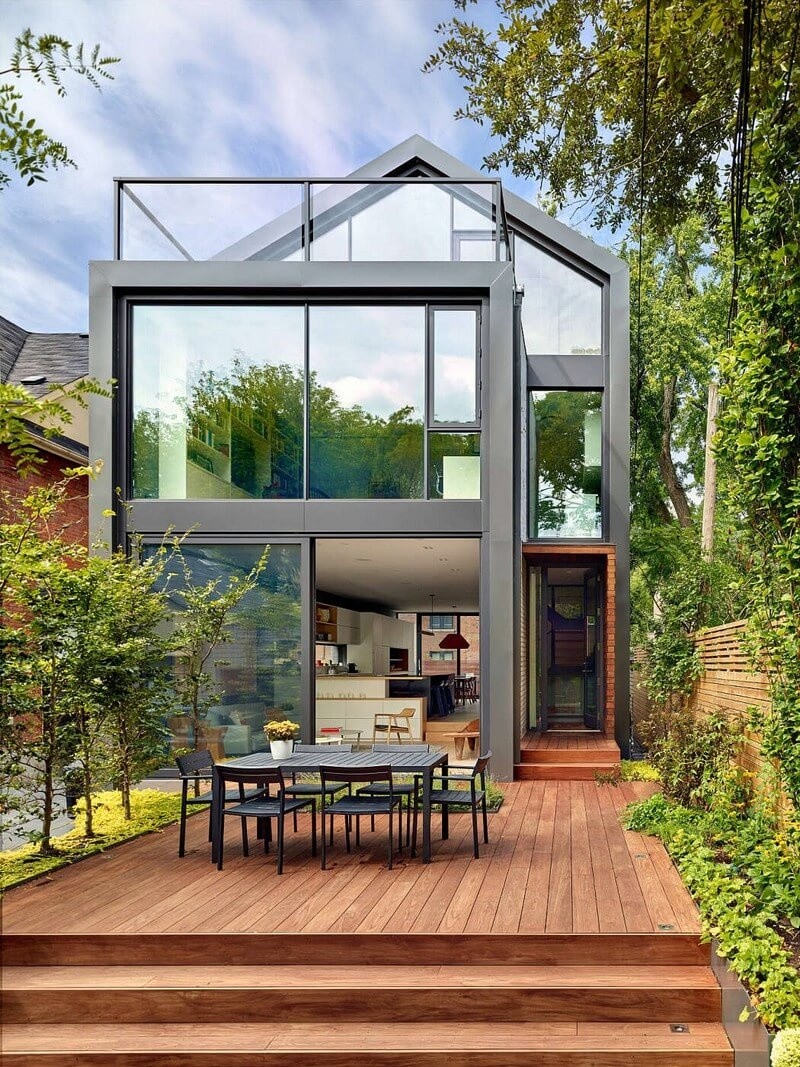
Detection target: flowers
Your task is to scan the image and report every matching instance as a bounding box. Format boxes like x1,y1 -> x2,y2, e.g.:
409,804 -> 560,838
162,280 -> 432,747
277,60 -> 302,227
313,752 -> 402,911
263,719 -> 300,743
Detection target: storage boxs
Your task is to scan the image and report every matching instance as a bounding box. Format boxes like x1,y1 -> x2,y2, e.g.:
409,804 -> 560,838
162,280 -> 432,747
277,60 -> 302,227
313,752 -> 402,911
318,608 -> 330,622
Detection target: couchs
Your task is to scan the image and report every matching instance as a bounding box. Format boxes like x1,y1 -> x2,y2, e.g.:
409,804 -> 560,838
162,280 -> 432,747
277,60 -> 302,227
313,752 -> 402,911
205,702 -> 292,754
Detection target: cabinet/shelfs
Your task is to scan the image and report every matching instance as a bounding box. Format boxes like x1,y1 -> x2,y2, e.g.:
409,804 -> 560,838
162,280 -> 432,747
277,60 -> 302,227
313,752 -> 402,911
315,602 -> 428,743
187,379 -> 300,499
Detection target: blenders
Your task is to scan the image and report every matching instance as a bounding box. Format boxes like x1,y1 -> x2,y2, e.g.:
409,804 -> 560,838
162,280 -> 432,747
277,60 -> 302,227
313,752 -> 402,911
316,660 -> 322,674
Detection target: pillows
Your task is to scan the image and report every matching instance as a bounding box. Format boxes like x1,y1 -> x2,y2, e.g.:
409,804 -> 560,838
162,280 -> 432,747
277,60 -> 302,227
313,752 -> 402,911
218,711 -> 244,725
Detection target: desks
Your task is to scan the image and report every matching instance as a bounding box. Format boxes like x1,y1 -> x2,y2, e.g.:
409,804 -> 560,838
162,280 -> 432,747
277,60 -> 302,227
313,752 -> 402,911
212,752 -> 448,864
315,729 -> 363,750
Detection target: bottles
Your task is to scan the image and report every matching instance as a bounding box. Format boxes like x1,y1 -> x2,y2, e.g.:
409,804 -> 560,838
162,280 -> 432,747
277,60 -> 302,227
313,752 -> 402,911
329,667 -> 336,674
326,632 -> 332,642
318,632 -> 324,642
324,663 -> 328,674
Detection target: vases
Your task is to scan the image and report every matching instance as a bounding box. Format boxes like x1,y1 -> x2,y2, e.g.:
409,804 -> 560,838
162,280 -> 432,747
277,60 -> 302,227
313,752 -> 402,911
270,740 -> 293,760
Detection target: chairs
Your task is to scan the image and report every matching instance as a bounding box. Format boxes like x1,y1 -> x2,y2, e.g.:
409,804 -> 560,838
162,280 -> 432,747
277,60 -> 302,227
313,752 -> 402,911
175,744 -> 492,875
373,708 -> 416,745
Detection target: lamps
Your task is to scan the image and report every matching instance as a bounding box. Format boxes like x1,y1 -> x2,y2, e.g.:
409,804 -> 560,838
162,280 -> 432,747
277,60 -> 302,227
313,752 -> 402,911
418,594 -> 435,636
439,606 -> 470,649
